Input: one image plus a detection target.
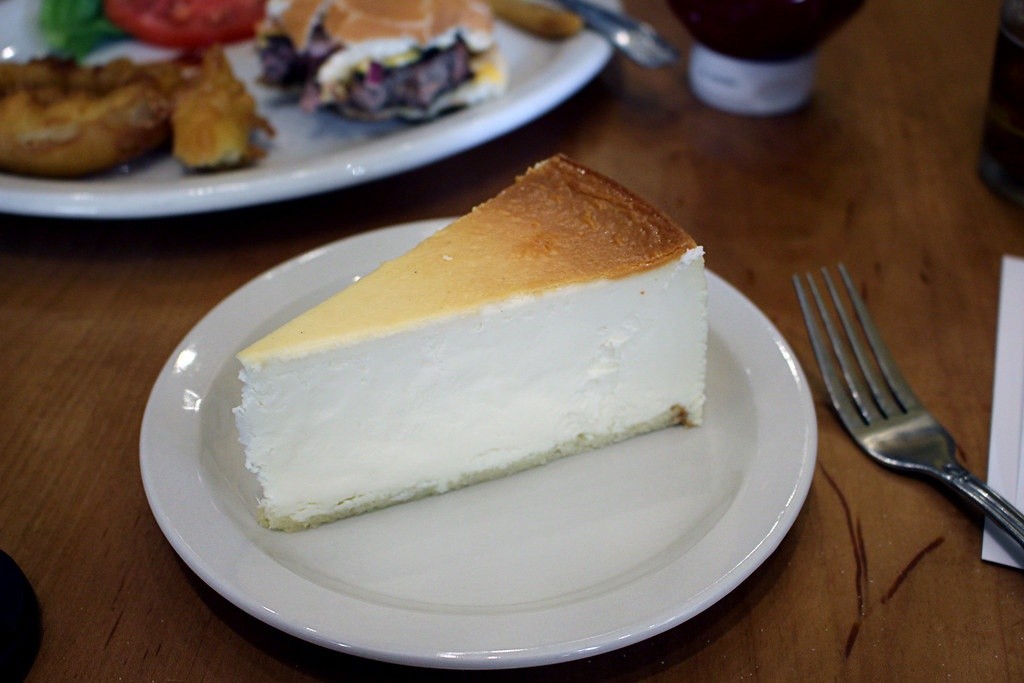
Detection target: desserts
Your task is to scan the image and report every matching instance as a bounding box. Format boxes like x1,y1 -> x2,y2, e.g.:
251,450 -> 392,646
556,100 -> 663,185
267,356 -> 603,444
235,154 -> 714,531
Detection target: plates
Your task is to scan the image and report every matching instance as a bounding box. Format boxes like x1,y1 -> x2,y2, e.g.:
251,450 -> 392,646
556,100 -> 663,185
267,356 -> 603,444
132,211 -> 816,667
2,0 -> 617,222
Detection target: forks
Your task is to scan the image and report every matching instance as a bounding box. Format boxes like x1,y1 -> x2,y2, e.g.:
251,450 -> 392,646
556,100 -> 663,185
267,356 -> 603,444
787,262 -> 1024,563
